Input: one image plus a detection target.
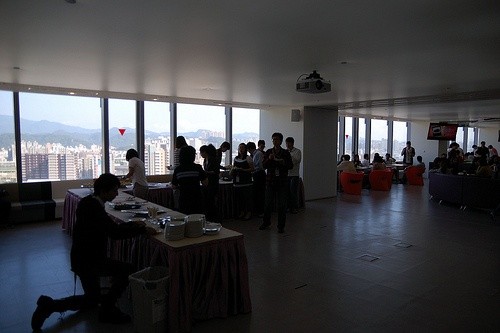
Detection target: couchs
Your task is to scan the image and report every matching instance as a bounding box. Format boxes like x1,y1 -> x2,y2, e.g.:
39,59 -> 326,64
371,168 -> 394,192
340,171 -> 364,195
406,166 -> 424,185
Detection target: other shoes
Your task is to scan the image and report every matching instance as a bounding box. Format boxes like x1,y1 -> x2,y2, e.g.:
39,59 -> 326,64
236,214 -> 251,221
278,228 -> 284,232
259,222 -> 271,230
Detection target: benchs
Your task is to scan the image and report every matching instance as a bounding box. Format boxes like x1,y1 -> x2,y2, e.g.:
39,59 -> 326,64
429,172 -> 500,216
0,179 -> 93,223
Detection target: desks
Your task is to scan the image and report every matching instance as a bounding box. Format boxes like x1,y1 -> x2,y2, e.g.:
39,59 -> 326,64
356,166 -> 370,170
392,163 -> 405,184
62,178 -> 305,333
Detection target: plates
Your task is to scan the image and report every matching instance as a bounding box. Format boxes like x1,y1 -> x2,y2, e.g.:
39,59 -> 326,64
164,221 -> 185,240
184,214 -> 206,238
274,158 -> 283,161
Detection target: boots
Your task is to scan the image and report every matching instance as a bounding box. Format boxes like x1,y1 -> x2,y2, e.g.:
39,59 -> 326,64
98,298 -> 132,324
31,294 -> 65,331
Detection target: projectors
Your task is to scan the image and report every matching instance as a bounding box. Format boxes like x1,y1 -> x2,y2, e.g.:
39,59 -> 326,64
296,79 -> 331,93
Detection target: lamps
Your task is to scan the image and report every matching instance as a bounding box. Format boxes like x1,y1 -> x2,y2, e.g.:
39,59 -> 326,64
291,109 -> 301,122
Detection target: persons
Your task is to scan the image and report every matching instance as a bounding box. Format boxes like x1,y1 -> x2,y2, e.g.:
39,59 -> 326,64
372,153 -> 386,165
351,154 -> 365,167
402,141 -> 415,165
167,136 -> 188,171
434,141 -> 500,208
337,155 -> 357,173
232,140 -> 267,220
257,133 -> 294,234
31,173 -> 148,332
386,153 -> 399,182
171,146 -> 209,216
122,149 -> 147,200
284,137 -> 301,214
362,154 -> 370,167
400,156 -> 426,184
200,142 -> 231,222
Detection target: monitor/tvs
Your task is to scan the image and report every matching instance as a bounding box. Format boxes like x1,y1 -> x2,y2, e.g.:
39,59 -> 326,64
427,123 -> 457,140
